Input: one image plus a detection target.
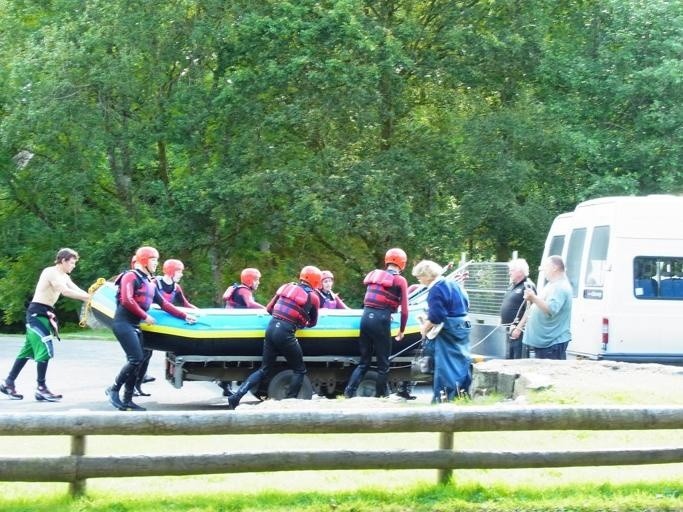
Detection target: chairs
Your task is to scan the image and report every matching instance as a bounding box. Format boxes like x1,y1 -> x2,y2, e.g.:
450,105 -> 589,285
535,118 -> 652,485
634,278 -> 683,299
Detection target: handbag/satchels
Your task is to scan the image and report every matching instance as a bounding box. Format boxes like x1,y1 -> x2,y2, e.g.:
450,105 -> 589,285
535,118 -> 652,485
91,280 -> 119,327
411,355 -> 434,377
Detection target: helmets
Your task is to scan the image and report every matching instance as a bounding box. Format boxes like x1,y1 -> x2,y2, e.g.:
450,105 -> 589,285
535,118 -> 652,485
299,264 -> 335,289
384,247 -> 408,264
162,258 -> 184,278
240,267 -> 262,288
130,247 -> 159,270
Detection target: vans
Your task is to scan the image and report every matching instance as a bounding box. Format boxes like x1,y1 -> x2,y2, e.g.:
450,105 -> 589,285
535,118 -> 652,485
532,193 -> 683,366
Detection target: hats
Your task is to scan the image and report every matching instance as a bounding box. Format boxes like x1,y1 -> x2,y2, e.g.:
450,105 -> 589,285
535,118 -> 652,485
411,260 -> 444,277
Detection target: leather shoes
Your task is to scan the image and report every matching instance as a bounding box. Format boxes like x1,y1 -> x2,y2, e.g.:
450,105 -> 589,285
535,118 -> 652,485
35,384 -> 61,401
0,381 -> 24,399
396,389 -> 417,400
220,382 -> 241,409
104,374 -> 155,410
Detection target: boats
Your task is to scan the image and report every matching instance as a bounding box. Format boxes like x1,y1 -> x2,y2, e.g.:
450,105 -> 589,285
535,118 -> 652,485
84,275 -> 462,357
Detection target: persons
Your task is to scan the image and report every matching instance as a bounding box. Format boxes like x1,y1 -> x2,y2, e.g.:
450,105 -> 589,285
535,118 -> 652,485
413,258 -> 473,403
314,269 -> 351,309
127,255 -> 151,396
343,246 -> 408,398
104,245 -> 199,410
0,247 -> 90,402
498,257 -> 537,359
216,267 -> 267,397
227,265 -> 324,410
396,260 -> 455,400
142,258 -> 198,383
522,254 -> 572,361
